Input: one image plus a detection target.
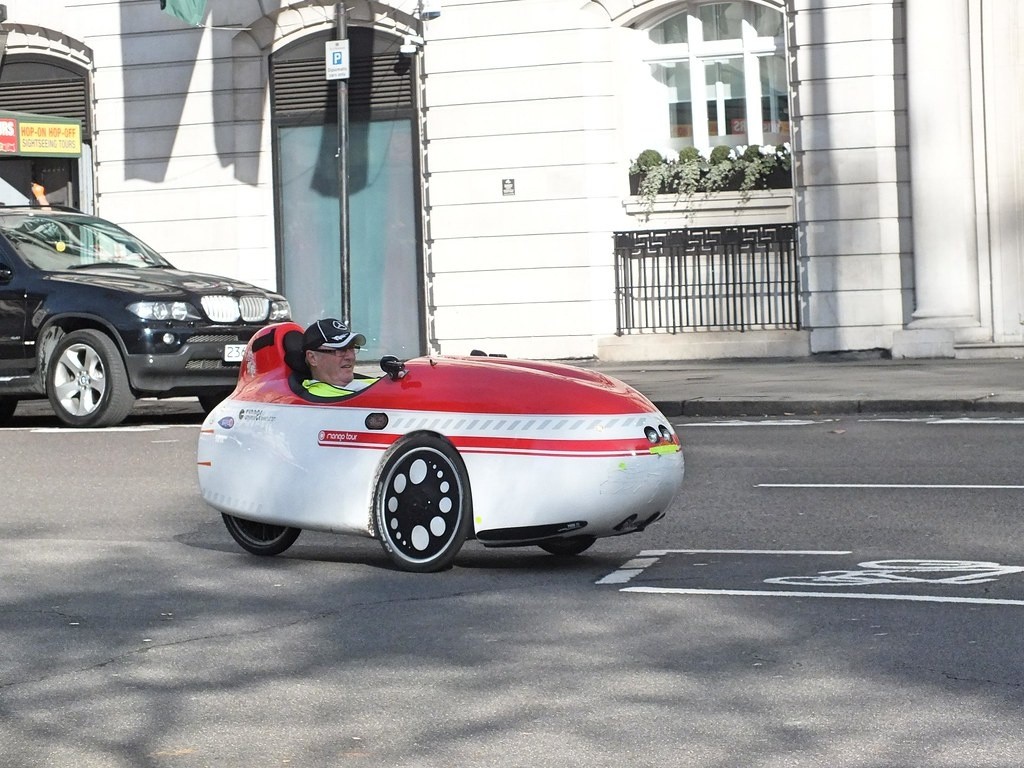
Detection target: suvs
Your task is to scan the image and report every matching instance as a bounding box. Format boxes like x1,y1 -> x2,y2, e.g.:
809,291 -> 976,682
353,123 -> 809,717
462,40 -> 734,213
0,201 -> 292,428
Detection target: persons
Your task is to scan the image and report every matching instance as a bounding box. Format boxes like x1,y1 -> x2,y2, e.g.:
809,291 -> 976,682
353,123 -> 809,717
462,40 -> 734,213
301,318 -> 382,397
31,183 -> 52,211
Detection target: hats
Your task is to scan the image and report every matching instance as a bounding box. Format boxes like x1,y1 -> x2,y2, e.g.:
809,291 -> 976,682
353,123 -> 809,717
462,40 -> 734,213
303,318 -> 366,351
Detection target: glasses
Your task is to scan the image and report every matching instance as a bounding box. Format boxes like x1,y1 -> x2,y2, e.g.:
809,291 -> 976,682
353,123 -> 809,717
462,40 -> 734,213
312,346 -> 361,356
